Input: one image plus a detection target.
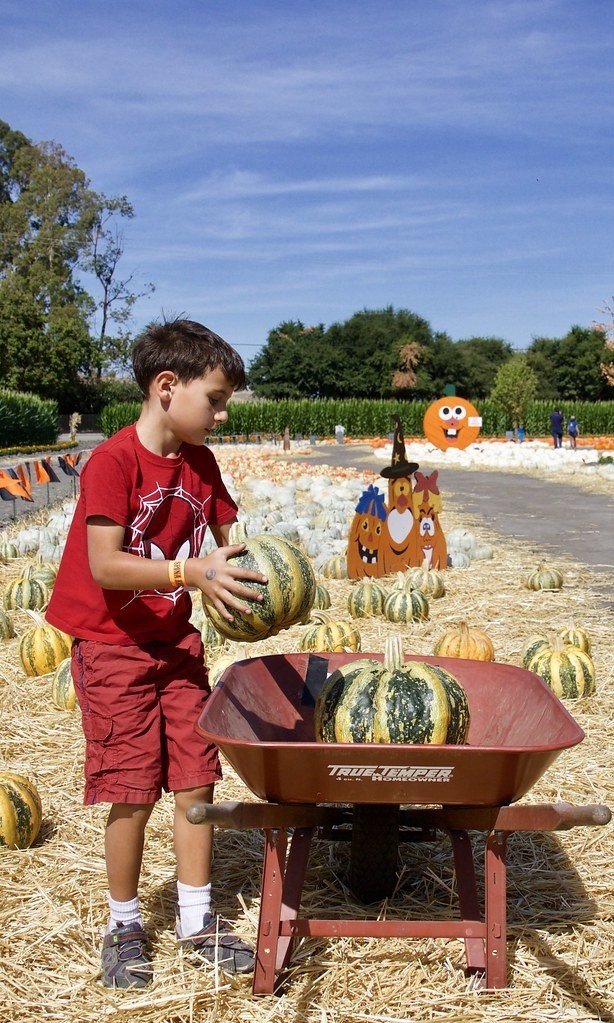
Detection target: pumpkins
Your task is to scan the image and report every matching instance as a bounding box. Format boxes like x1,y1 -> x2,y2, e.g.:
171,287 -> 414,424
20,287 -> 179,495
0,773 -> 43,851
1,443 -> 78,709
186,435 -> 614,699
314,659 -> 471,745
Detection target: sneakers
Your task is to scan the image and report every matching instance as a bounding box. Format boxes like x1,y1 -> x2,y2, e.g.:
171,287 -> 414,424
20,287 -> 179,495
174,910 -> 255,974
100,916 -> 154,990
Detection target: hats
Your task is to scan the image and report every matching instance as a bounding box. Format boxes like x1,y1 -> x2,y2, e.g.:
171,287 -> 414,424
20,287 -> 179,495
570,415 -> 576,419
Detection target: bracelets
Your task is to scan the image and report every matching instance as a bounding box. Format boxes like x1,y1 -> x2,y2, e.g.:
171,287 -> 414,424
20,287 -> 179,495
169,559 -> 189,587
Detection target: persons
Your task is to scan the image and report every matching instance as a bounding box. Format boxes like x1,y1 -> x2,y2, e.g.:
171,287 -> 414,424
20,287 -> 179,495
549,407 -> 565,448
45,319 -> 267,991
567,415 -> 579,448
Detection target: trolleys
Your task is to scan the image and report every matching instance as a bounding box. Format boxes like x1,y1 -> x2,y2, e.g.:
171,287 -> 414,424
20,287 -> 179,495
185,652 -> 612,997
